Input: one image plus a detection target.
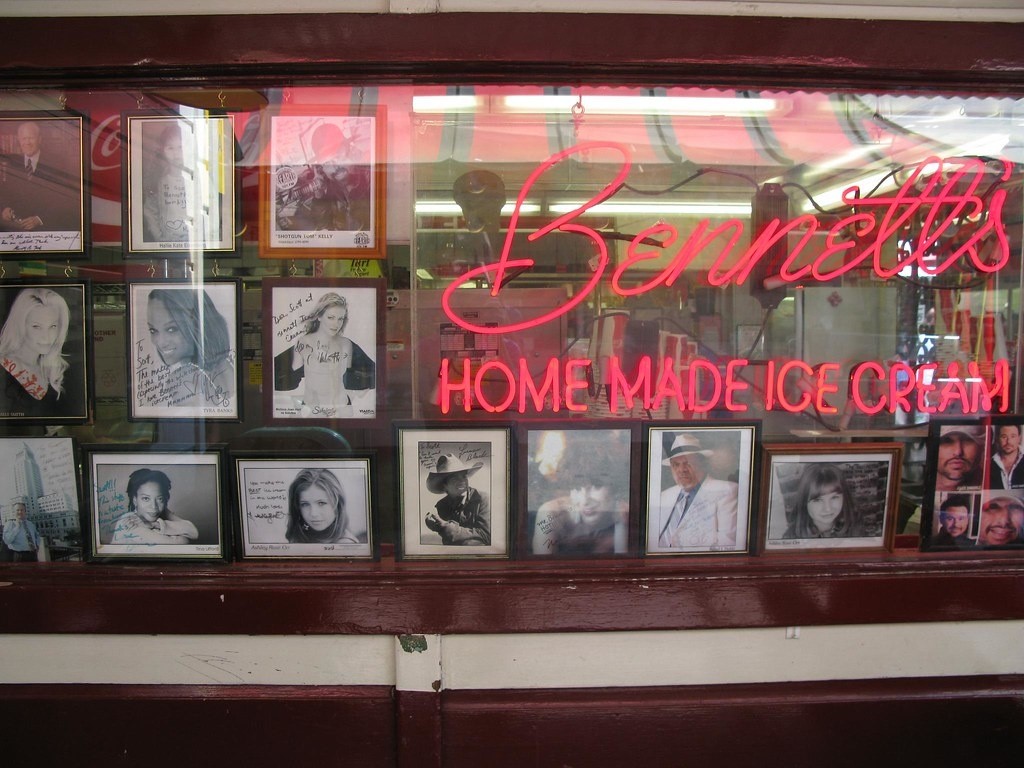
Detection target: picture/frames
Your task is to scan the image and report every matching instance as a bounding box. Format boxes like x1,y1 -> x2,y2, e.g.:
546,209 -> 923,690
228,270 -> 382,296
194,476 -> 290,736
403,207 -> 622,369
393,418 -> 518,560
124,278 -> 245,424
0,107 -> 92,261
119,107 -> 244,260
518,420 -> 640,558
637,418 -> 763,558
229,448 -> 382,563
0,435 -> 88,562
757,440 -> 905,554
261,276 -> 388,430
258,103 -> 388,258
0,275 -> 96,426
917,414 -> 1024,550
78,443 -> 235,567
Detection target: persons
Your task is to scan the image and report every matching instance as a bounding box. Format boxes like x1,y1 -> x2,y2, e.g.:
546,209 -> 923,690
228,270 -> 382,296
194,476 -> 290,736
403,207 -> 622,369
990,425 -> 1024,490
3,503 -> 41,563
424,453 -> 490,547
932,499 -> 975,547
936,424 -> 983,490
142,124 -> 220,242
978,492 -> 1024,547
0,286 -> 71,415
0,120 -> 61,231
783,462 -> 867,540
280,124 -> 370,231
273,293 -> 376,406
146,289 -> 236,406
111,470 -> 200,544
532,441 -> 628,555
657,433 -> 738,544
285,469 -> 360,544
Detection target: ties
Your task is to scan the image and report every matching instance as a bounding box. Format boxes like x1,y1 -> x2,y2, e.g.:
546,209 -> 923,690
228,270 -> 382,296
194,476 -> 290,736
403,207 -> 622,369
22,521 -> 36,551
24,158 -> 33,179
657,491 -> 688,548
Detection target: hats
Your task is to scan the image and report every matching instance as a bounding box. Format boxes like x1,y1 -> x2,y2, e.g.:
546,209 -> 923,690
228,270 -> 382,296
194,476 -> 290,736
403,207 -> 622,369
306,123 -> 361,161
982,489 -> 1024,506
941,425 -> 986,446
665,432 -> 714,460
425,453 -> 482,494
